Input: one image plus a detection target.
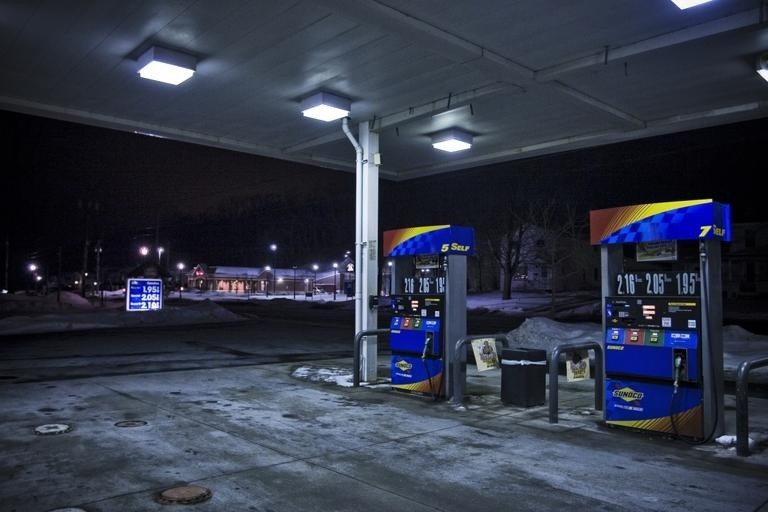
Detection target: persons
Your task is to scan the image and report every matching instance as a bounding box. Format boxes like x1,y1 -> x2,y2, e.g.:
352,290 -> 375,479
482,340 -> 497,368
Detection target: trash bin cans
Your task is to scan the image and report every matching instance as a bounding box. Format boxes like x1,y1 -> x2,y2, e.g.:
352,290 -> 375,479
500,347 -> 547,408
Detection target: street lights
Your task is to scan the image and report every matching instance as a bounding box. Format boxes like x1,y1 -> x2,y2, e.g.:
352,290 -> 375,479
136,244 -> 186,305
94,245 -> 101,294
262,242 -> 393,302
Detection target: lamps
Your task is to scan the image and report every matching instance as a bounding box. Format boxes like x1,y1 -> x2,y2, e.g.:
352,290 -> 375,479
299,92 -> 352,123
135,46 -> 198,87
431,129 -> 473,153
